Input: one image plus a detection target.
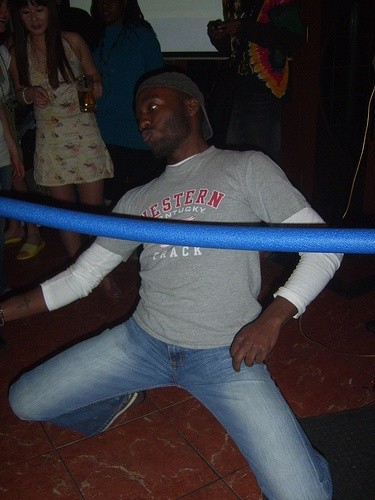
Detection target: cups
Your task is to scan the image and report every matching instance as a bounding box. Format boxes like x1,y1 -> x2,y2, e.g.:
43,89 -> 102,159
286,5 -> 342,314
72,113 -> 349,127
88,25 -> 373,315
75,73 -> 96,112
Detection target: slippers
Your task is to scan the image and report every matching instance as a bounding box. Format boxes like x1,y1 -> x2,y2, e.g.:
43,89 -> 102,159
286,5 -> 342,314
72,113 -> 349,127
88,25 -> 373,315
5,237 -> 22,245
16,242 -> 46,259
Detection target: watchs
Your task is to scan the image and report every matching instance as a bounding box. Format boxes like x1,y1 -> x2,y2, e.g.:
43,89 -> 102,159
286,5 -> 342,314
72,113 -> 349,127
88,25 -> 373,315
0,308 -> 6,327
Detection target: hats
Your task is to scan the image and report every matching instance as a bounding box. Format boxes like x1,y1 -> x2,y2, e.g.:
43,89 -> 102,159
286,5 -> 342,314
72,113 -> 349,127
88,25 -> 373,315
137,72 -> 213,139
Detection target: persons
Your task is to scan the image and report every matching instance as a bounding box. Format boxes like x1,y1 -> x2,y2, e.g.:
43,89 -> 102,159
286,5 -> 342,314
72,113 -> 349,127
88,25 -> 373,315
0,0 -> 309,264
0,72 -> 344,500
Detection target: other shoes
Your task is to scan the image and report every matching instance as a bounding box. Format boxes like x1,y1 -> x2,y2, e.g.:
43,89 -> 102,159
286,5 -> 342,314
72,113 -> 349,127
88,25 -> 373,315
103,273 -> 125,299
100,391 -> 144,433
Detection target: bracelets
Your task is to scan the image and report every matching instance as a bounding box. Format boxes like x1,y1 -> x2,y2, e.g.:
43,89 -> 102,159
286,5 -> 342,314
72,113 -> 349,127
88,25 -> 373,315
22,86 -> 33,105
